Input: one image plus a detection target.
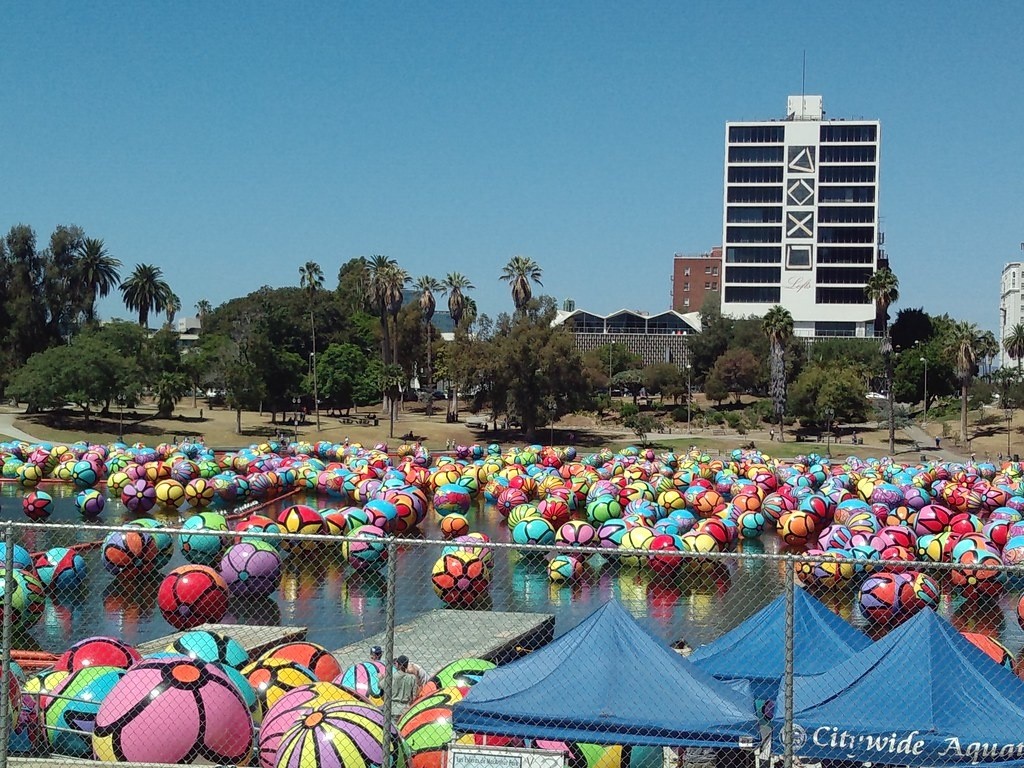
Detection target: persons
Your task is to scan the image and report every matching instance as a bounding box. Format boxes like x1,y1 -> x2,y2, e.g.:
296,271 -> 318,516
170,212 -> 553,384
446,439 -> 451,450
199,435 -> 204,444
935,436 -> 941,447
192,437 -> 196,444
452,439 -> 457,451
409,431 -> 413,437
172,436 -> 177,445
275,427 -> 291,446
483,423 -> 488,432
392,658 -> 431,686
344,436 -> 349,446
200,409 -> 203,418
448,411 -> 458,423
302,413 -> 305,421
293,413 -> 297,421
367,413 -> 376,419
997,452 -> 1002,461
288,417 -> 292,422
299,412 -> 303,421
770,429 -> 774,440
851,431 -> 858,445
377,655 -> 418,724
183,435 -> 189,443
370,645 -> 384,663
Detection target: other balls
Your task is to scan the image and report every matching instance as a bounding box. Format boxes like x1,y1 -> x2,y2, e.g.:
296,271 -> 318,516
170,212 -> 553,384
0,436 -> 1024,767
